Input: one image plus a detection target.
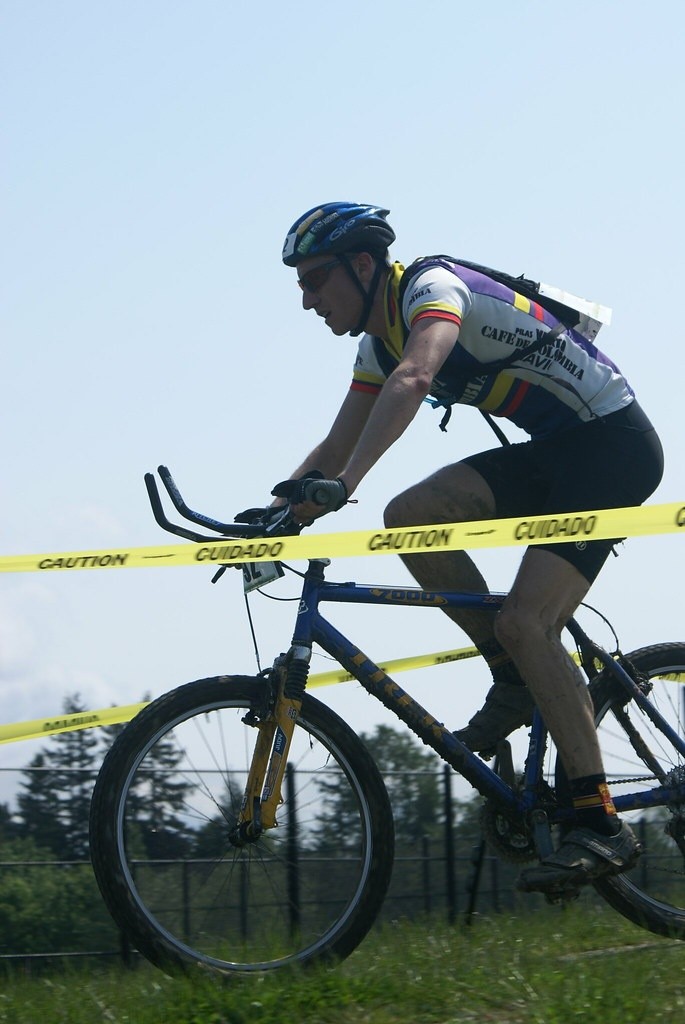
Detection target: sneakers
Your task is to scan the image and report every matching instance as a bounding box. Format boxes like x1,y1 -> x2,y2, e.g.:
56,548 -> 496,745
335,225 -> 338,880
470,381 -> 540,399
520,819 -> 641,886
453,681 -> 536,752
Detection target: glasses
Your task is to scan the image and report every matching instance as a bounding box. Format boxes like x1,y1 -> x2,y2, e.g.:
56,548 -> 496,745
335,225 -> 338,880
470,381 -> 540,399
297,255 -> 358,292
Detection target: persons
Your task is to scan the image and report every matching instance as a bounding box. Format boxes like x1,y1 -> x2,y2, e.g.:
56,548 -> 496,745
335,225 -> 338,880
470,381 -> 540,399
265,202 -> 664,895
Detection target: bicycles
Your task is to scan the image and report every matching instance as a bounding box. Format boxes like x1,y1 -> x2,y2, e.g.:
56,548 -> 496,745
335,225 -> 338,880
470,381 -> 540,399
89,465 -> 684,995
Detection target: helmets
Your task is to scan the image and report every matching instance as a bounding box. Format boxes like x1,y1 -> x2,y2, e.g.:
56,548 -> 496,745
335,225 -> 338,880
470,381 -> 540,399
282,203 -> 396,267
